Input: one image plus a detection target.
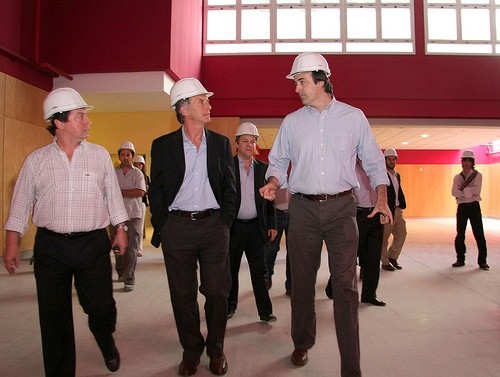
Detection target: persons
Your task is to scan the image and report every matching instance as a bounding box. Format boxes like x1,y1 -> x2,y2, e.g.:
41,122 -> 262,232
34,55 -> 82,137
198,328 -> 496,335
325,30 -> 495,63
380,147 -> 407,270
143,77 -> 242,377
259,52 -> 393,377
226,121 -> 277,323
452,150 -> 491,269
2,89 -> 130,377
325,157 -> 387,307
269,163 -> 292,295
108,140 -> 151,292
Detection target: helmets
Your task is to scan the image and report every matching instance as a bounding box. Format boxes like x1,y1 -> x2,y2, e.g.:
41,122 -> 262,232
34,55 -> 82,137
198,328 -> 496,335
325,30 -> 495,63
118,141 -> 136,153
169,77 -> 214,108
285,52 -> 332,79
42,87 -> 94,122
460,150 -> 474,159
234,122 -> 259,138
133,155 -> 145,164
383,147 -> 398,157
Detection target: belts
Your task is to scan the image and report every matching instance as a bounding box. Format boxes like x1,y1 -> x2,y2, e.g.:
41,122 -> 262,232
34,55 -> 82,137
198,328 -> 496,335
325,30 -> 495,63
295,186 -> 353,203
38,226 -> 100,241
169,207 -> 219,221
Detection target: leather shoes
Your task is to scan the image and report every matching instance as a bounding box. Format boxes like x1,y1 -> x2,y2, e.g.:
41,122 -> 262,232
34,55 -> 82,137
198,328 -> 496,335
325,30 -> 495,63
209,353 -> 228,376
290,348 -> 308,366
178,356 -> 200,377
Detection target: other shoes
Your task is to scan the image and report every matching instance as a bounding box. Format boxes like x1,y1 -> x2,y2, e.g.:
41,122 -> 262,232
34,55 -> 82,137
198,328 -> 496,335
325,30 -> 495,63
124,284 -> 134,292
118,275 -> 125,282
360,297 -> 385,306
228,310 -> 235,319
452,262 -> 465,267
263,314 -> 277,323
100,338 -> 121,372
479,264 -> 489,269
388,258 -> 402,269
325,283 -> 333,300
382,264 -> 394,271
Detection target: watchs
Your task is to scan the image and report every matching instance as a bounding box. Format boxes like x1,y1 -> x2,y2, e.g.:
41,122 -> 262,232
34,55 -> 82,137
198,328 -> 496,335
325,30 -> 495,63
118,222 -> 129,232
